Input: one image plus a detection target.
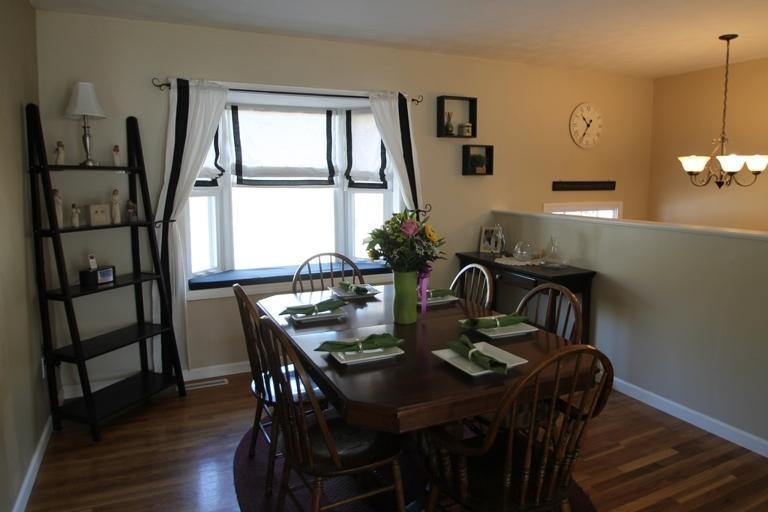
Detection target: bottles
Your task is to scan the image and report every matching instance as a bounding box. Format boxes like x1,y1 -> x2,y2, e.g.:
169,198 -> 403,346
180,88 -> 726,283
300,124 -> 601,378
462,120 -> 472,137
487,223 -> 566,269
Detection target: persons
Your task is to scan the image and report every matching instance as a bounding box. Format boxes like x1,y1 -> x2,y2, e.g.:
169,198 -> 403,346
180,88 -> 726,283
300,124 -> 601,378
53,188 -> 64,230
54,138 -> 65,164
71,202 -> 81,228
112,145 -> 121,167
111,188 -> 122,224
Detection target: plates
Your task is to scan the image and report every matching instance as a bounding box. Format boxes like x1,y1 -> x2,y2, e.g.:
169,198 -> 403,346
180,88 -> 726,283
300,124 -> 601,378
418,296 -> 458,307
433,341 -> 531,376
328,283 -> 381,301
457,313 -> 538,340
321,335 -> 406,366
292,308 -> 349,325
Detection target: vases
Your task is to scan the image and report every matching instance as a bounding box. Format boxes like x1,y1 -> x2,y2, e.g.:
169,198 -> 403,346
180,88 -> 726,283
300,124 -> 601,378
388,270 -> 421,325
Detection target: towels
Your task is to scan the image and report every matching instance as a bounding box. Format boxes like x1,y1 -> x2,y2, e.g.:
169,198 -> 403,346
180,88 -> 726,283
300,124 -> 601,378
456,309 -> 532,340
414,286 -> 459,304
335,278 -> 369,297
274,291 -> 352,318
445,334 -> 508,383
309,329 -> 407,356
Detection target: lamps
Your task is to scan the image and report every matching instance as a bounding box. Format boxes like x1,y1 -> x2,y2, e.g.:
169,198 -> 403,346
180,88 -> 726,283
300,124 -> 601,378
58,78 -> 111,167
673,31 -> 767,191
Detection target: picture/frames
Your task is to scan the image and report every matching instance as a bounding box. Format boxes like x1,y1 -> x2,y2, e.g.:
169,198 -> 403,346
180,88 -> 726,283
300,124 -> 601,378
477,224 -> 506,256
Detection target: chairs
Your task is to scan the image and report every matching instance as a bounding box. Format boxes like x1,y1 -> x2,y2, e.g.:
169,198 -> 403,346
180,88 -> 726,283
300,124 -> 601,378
447,259 -> 494,309
287,252 -> 364,298
229,282 -> 326,463
462,274 -> 584,437
249,304 -> 407,511
460,342 -> 615,512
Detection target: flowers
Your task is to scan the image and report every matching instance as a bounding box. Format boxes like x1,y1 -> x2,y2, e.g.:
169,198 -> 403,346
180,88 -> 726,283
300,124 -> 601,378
364,198 -> 447,275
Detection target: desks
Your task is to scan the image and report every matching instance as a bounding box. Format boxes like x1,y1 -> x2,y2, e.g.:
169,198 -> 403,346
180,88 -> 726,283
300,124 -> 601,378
455,249 -> 600,351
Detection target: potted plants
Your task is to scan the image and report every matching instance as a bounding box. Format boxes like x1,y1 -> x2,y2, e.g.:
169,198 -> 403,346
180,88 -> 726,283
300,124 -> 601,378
470,153 -> 486,175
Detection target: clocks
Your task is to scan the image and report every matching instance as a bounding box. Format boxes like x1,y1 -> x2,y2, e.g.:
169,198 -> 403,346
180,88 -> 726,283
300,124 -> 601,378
568,100 -> 604,149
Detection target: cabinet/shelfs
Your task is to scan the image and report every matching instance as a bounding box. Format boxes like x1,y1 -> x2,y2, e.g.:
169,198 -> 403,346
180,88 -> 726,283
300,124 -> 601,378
435,94 -> 493,176
19,101 -> 187,442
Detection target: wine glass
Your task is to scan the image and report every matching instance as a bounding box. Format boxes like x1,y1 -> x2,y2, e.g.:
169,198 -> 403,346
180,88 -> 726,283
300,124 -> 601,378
445,121 -> 455,135
